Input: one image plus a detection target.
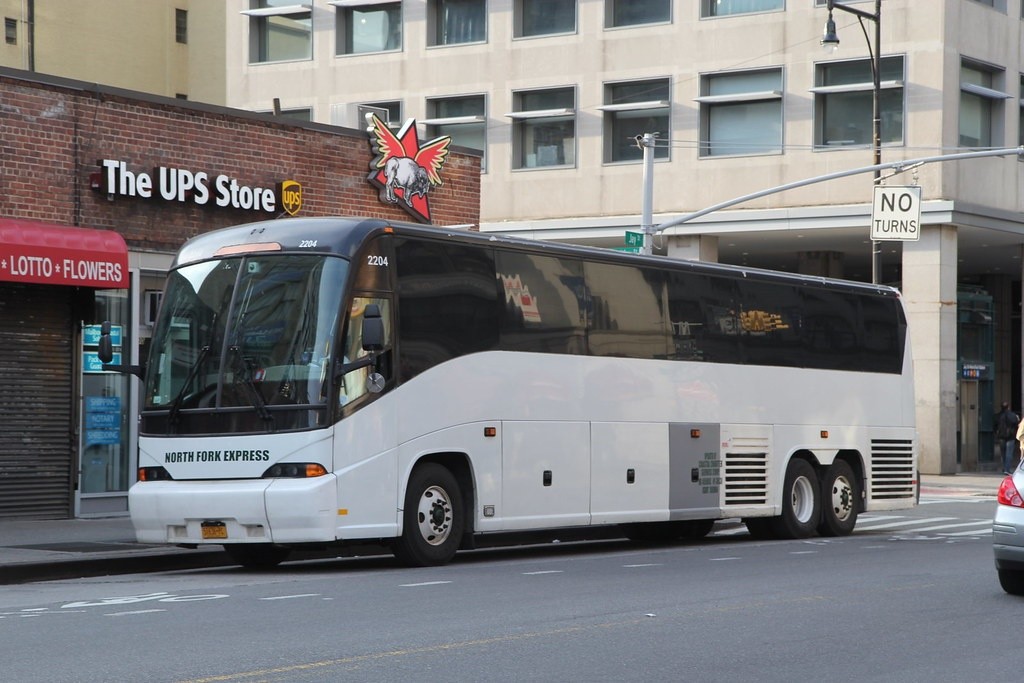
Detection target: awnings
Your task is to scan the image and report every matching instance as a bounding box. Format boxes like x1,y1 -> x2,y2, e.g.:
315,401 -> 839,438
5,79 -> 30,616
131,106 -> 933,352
0,217 -> 129,289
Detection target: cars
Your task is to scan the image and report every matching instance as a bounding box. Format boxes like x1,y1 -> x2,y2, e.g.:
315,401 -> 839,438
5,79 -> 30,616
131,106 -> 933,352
992,455 -> 1024,596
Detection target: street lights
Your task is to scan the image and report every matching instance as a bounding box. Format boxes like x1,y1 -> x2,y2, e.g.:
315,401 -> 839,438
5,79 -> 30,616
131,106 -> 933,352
819,1 -> 881,283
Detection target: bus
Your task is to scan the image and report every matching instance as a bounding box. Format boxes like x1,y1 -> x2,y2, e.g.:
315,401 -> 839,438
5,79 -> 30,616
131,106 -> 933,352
98,217 -> 920,570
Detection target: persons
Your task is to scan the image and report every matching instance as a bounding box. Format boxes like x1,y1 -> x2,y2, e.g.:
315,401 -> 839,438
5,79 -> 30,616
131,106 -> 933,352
1016,417 -> 1024,461
993,402 -> 1020,475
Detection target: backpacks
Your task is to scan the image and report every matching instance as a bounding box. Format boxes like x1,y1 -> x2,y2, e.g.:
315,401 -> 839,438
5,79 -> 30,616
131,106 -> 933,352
995,410 -> 1013,440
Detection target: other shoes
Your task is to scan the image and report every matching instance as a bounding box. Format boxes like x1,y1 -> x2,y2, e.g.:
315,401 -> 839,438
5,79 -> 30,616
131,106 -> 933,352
1004,468 -> 1013,475
